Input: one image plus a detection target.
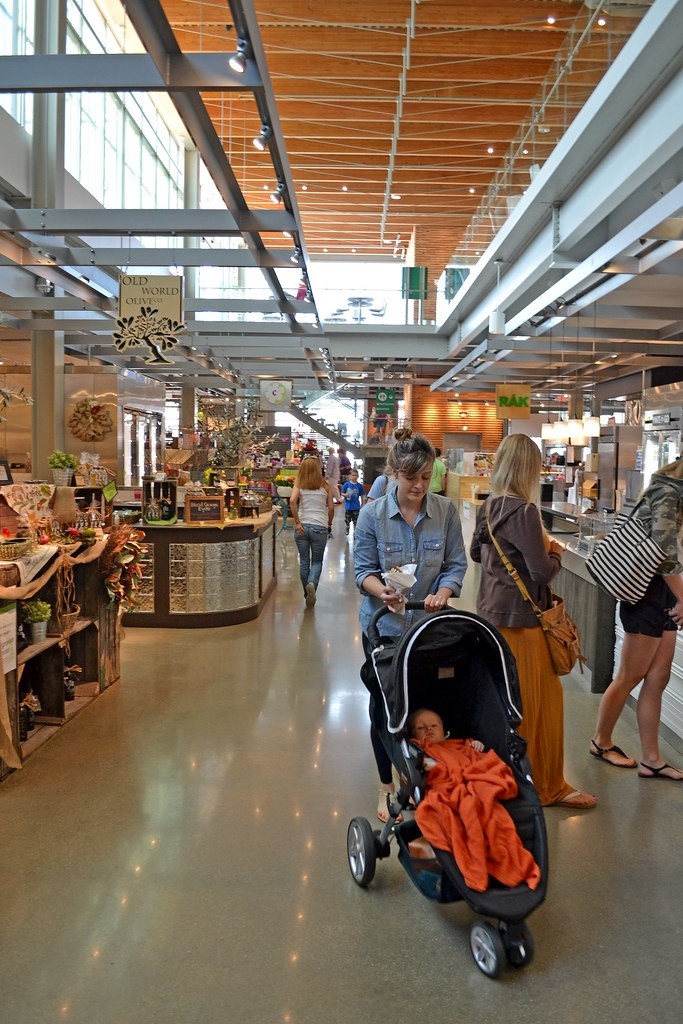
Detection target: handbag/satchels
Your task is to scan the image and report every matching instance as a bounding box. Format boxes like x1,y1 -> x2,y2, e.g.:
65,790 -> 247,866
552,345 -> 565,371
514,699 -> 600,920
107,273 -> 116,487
584,480 -> 680,605
536,593 -> 588,676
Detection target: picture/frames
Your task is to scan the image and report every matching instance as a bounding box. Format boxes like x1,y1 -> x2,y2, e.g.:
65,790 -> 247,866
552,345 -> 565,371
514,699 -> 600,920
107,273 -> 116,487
186,496 -> 225,524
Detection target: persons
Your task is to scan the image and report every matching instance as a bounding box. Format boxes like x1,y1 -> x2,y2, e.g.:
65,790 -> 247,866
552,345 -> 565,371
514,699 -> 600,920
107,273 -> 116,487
589,449 -> 683,780
324,448 -> 351,507
293,431 -> 317,450
341,468 -> 365,535
412,709 -> 485,768
470,433 -> 599,809
290,458 -> 334,609
428,447 -> 447,496
374,410 -> 390,436
353,425 -> 468,828
366,470 -> 399,505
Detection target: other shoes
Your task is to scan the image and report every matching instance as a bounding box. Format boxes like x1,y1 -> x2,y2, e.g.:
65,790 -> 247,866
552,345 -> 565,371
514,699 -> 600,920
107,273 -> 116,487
345,526 -> 349,536
305,582 -> 316,607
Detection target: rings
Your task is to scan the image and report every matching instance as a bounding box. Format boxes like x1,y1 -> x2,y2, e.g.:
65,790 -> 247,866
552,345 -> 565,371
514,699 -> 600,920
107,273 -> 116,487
436,602 -> 438,604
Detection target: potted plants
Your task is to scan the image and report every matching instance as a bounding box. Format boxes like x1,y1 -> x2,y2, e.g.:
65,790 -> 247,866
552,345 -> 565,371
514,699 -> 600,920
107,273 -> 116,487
46,450 -> 69,487
23,598 -> 51,644
64,454 -> 76,486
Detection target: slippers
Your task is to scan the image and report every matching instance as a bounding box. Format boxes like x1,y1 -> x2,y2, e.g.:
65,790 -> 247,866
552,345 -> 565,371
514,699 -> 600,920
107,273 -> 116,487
550,788 -> 597,808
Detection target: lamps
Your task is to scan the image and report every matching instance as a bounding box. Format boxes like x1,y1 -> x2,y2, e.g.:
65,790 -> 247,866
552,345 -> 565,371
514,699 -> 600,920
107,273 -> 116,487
312,321 -> 319,328
229,38 -> 251,73
283,231 -> 293,237
290,247 -> 301,263
270,182 -> 286,204
489,260 -> 505,334
304,290 -> 312,303
541,300 -> 601,440
319,347 -> 335,389
253,123 -> 272,151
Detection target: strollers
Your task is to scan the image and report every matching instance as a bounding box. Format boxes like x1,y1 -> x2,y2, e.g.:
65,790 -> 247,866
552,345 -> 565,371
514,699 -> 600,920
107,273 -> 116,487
346,600 -> 549,980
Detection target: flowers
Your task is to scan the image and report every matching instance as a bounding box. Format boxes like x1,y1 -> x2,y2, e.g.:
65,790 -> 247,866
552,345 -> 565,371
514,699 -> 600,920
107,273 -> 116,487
274,475 -> 295,488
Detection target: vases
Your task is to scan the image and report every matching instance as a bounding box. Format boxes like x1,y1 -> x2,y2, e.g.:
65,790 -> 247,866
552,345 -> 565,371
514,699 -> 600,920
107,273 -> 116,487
276,486 -> 293,497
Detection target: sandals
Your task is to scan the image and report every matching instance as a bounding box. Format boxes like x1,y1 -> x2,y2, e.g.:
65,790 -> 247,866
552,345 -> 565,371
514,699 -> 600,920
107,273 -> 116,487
638,759 -> 683,780
589,738 -> 638,767
377,787 -> 401,825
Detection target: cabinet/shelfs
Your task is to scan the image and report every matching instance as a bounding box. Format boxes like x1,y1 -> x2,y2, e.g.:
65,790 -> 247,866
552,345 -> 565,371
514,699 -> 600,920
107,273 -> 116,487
0,534 -> 101,781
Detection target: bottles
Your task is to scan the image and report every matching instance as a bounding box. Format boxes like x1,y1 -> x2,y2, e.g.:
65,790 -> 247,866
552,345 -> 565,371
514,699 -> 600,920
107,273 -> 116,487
346,488 -> 351,500
232,508 -> 238,519
89,467 -> 108,487
